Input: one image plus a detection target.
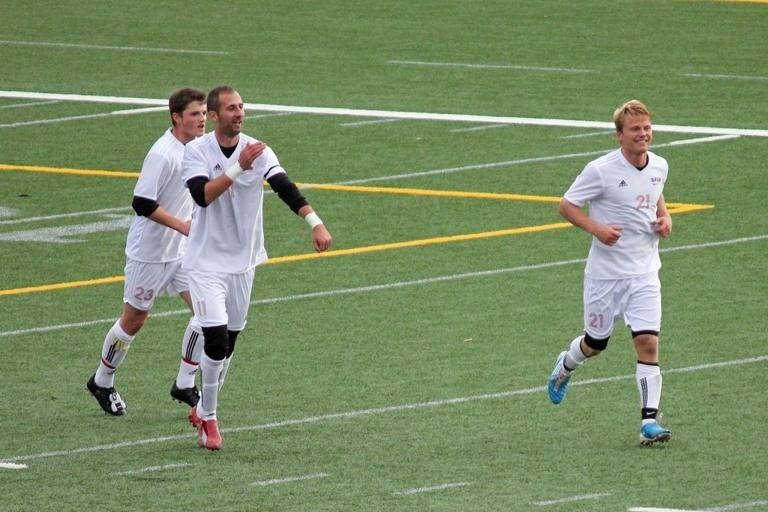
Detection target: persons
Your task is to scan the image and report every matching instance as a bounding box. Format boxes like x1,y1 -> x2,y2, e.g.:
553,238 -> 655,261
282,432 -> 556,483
546,99 -> 671,446
87,89 -> 210,417
177,87 -> 331,452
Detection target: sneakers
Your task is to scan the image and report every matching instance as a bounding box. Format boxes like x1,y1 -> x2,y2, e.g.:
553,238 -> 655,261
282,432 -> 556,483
640,422 -> 671,445
86,373 -> 127,416
170,380 -> 222,451
548,350 -> 576,404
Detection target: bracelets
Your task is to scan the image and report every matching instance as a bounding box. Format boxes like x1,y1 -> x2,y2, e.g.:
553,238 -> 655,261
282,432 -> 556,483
225,162 -> 243,180
305,211 -> 323,230
